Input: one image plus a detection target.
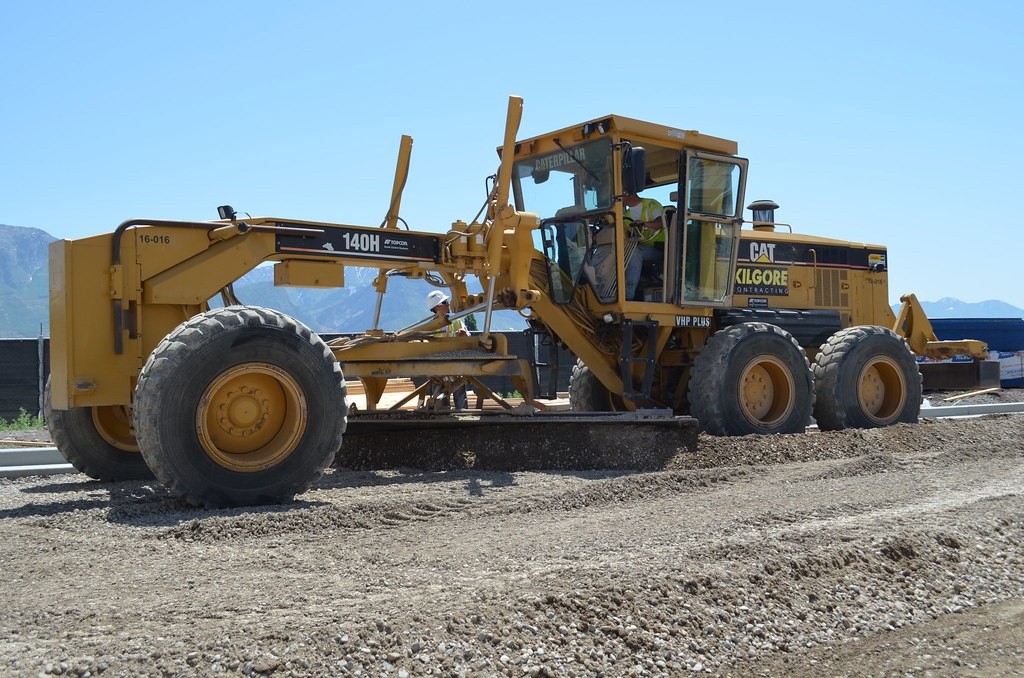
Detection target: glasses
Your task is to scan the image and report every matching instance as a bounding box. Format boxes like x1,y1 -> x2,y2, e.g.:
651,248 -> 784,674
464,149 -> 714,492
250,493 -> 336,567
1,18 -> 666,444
439,301 -> 449,306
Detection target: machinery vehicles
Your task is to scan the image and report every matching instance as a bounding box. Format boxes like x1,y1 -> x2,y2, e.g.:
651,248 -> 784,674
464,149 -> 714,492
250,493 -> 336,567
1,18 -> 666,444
39,94 -> 1003,513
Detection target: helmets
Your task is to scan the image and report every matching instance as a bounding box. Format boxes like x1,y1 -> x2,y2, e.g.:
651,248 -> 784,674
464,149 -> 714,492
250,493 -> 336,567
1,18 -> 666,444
427,291 -> 449,309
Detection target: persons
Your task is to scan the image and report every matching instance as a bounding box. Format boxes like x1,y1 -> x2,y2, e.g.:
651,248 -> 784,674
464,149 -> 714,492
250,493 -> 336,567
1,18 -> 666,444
427,291 -> 471,409
623,193 -> 667,301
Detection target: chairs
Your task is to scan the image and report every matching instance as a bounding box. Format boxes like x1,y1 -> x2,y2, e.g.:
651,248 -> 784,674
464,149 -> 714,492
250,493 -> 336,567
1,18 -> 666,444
642,205 -> 675,279
